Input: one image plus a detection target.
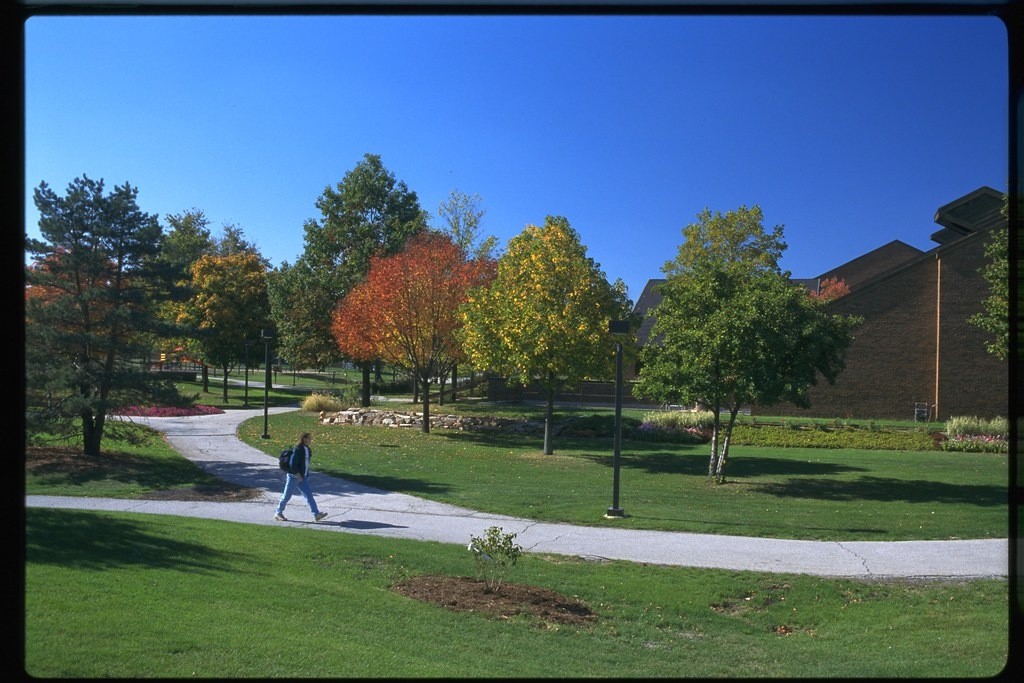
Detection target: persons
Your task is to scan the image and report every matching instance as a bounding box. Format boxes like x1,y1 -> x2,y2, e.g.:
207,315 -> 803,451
272,432 -> 327,521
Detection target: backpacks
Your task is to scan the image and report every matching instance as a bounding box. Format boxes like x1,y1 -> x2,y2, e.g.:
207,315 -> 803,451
279,445 -> 293,472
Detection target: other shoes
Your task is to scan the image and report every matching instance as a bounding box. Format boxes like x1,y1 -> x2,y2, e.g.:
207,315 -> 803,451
315,512 -> 327,521
274,513 -> 287,520
